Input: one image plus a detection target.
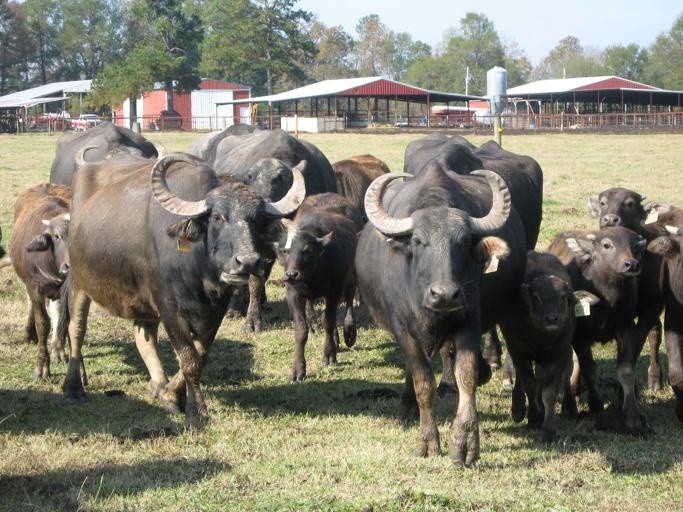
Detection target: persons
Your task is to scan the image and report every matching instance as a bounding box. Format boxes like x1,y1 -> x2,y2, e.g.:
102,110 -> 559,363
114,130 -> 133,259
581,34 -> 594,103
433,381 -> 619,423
419,116 -> 426,126
529,117 -> 535,129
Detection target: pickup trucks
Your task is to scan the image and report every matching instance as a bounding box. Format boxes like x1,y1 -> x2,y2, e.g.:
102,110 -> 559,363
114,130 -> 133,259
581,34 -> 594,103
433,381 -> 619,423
71,113 -> 102,132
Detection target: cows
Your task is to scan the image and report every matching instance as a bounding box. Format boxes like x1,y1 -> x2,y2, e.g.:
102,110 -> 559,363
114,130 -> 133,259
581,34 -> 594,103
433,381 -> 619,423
48,122 -> 163,188
11,183 -> 73,377
61,157 -> 305,437
199,125 -> 683,469
